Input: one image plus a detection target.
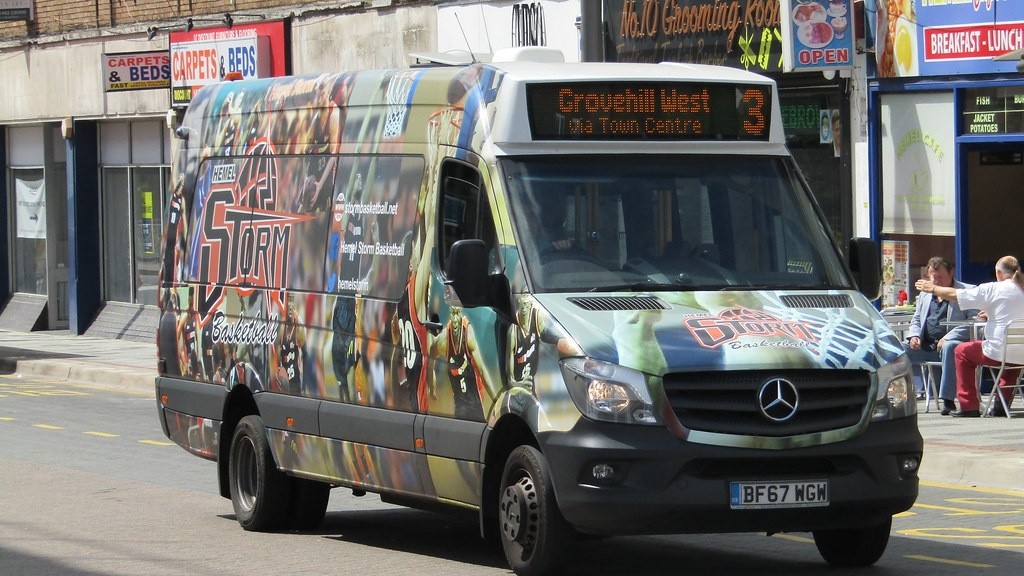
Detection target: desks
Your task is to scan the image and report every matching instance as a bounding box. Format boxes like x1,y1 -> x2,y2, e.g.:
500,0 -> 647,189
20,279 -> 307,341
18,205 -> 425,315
939,320 -> 992,415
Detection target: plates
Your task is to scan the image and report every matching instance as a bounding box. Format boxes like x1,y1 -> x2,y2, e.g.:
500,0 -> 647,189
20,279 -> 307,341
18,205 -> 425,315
827,8 -> 847,17
792,2 -> 827,27
797,20 -> 834,48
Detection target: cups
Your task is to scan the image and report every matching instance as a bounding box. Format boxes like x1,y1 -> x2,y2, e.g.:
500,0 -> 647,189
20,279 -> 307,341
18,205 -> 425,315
829,2 -> 846,13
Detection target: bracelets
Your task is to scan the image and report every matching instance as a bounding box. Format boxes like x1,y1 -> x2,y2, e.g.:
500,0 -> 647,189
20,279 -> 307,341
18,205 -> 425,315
933,285 -> 937,293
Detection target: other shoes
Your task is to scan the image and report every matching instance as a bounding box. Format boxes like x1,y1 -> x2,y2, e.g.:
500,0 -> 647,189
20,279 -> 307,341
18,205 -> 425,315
989,407 -> 1006,417
940,404 -> 957,415
949,409 -> 981,417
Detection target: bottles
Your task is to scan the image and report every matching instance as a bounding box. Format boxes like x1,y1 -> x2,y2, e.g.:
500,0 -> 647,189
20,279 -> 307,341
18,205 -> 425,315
899,290 -> 907,306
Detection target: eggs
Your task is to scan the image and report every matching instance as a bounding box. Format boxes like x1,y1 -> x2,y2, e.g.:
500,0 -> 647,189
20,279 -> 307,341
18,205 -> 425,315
892,15 -> 919,76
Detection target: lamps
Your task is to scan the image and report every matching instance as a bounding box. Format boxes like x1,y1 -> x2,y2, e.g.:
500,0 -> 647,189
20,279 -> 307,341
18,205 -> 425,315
184,18 -> 226,32
221,13 -> 265,29
146,23 -> 187,40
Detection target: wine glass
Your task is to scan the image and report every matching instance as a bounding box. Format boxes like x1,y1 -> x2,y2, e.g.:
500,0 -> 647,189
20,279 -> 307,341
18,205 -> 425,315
831,17 -> 847,39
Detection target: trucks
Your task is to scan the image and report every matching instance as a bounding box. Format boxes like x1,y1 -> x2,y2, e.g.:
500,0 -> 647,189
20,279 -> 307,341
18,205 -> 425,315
141,41 -> 922,576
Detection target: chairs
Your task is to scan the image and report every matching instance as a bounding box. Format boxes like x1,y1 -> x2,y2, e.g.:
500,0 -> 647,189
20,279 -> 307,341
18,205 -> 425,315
980,318 -> 1024,418
878,305 -> 916,333
910,360 -> 942,416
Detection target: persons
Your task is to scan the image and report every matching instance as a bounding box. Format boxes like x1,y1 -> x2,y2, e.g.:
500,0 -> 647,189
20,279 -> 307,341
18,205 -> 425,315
391,109 -> 454,413
903,256 -> 984,415
488,203 -> 571,287
915,255 -> 1024,418
428,306 -> 497,420
162,73 -> 340,397
506,284 -> 574,397
332,69 -> 394,405
831,112 -> 840,157
